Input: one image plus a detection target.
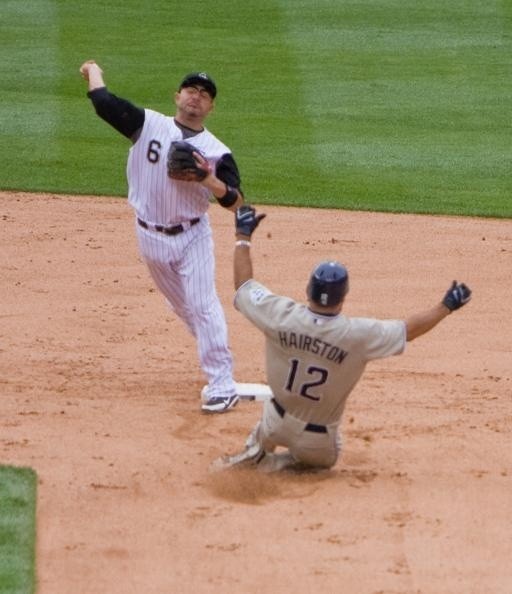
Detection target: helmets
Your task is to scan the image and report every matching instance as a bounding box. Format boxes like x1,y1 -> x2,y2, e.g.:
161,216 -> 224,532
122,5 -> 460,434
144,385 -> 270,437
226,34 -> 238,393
308,261 -> 349,307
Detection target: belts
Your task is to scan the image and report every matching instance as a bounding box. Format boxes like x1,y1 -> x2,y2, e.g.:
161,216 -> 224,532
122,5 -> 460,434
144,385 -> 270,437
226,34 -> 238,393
138,217 -> 200,236
271,398 -> 328,434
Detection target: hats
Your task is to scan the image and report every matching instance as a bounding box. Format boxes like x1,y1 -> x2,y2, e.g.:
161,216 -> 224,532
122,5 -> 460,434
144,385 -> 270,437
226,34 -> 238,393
179,73 -> 216,99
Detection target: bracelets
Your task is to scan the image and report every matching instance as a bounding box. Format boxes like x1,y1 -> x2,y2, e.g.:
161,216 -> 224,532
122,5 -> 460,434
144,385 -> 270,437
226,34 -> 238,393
212,184 -> 239,208
235,240 -> 251,248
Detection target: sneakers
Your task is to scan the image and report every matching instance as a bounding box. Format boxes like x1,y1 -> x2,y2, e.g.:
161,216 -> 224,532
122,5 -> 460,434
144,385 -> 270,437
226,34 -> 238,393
258,450 -> 298,474
207,441 -> 264,476
201,393 -> 239,413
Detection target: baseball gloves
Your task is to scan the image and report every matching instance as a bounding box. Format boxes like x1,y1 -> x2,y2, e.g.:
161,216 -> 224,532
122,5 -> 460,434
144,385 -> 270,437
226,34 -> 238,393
167,142 -> 208,183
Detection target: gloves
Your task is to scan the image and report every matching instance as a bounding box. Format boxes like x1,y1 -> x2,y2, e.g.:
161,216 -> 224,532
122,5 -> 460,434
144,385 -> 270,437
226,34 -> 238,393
235,205 -> 267,236
442,279 -> 472,311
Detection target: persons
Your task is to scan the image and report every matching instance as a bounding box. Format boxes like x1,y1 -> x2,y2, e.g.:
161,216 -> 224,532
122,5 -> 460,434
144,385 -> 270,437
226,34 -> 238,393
79,58 -> 244,412
209,204 -> 473,476
